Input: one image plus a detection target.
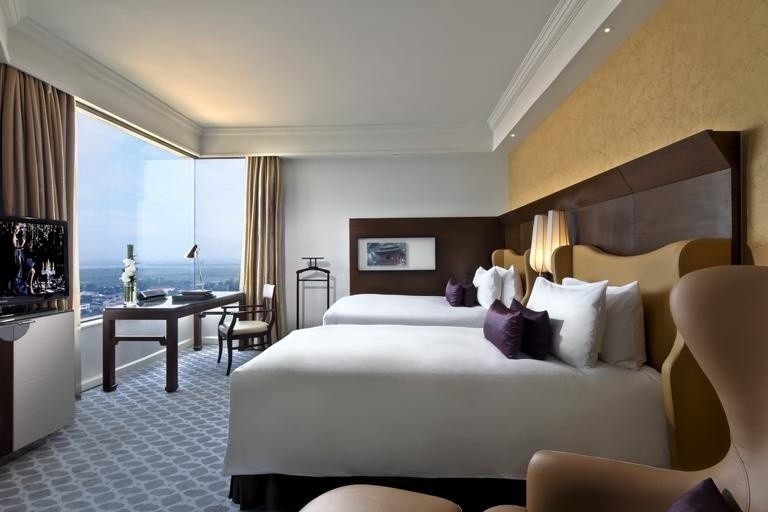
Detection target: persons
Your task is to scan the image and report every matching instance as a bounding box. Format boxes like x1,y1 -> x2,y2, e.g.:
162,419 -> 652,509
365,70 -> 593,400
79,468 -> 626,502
9,222 -> 36,294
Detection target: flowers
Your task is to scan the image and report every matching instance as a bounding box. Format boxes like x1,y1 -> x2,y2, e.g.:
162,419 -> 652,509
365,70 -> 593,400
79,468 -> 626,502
120,246 -> 137,300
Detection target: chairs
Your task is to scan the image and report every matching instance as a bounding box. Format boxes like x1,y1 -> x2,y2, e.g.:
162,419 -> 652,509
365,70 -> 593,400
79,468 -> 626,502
295,264 -> 768,510
217,284 -> 275,376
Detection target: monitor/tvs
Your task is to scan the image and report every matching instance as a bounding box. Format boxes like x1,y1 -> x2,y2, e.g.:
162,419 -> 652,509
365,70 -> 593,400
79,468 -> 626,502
0,215 -> 69,307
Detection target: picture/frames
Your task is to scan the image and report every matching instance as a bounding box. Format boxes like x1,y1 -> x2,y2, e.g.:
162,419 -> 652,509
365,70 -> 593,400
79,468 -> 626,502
355,235 -> 438,273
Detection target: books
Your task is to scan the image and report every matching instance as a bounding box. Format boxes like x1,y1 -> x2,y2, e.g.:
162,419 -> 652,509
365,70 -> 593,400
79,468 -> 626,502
172,289 -> 216,302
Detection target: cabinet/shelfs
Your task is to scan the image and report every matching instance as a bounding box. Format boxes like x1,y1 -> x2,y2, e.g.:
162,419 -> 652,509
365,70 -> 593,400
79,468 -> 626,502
0,318 -> 14,459
13,311 -> 74,454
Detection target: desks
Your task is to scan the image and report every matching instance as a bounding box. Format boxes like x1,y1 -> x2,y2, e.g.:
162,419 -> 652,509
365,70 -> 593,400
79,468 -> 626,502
103,291 -> 247,392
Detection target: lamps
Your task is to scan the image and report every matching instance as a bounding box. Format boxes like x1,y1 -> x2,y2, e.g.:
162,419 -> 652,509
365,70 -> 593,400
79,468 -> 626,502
529,214 -> 548,278
546,211 -> 568,274
186,242 -> 210,301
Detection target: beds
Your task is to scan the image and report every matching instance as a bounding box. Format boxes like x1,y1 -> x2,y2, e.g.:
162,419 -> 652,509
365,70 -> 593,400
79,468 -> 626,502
322,248 -> 543,325
226,235 -> 730,511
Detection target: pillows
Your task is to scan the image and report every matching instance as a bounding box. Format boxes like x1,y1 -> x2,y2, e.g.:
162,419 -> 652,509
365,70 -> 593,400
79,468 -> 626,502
443,278 -> 462,306
470,266 -> 500,308
463,280 -> 476,306
483,300 -> 523,360
526,278 -> 609,370
495,265 -> 524,308
562,278 -> 646,371
509,298 -> 551,360
654,479 -> 741,512
